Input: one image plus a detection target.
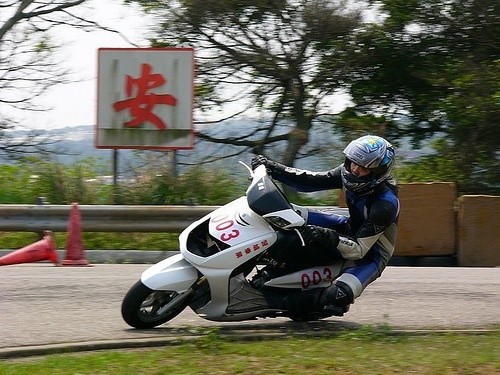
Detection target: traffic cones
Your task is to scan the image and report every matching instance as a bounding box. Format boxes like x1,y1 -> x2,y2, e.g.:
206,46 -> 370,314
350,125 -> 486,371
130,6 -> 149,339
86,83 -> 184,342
59,202 -> 90,265
0,230 -> 59,265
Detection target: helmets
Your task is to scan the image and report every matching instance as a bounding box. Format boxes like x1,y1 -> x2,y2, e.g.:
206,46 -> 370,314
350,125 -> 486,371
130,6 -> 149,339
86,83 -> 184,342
341,136 -> 395,190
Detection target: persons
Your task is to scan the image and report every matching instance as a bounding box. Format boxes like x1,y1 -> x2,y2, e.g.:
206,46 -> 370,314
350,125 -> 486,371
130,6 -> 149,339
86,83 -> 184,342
252,135 -> 401,317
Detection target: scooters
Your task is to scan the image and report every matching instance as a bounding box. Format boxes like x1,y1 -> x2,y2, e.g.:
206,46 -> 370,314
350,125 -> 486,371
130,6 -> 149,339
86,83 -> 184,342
121,153 -> 351,330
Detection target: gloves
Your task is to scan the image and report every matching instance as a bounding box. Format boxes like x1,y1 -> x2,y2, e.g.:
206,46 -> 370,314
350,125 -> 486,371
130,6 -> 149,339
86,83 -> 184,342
296,225 -> 339,249
251,155 -> 284,179
